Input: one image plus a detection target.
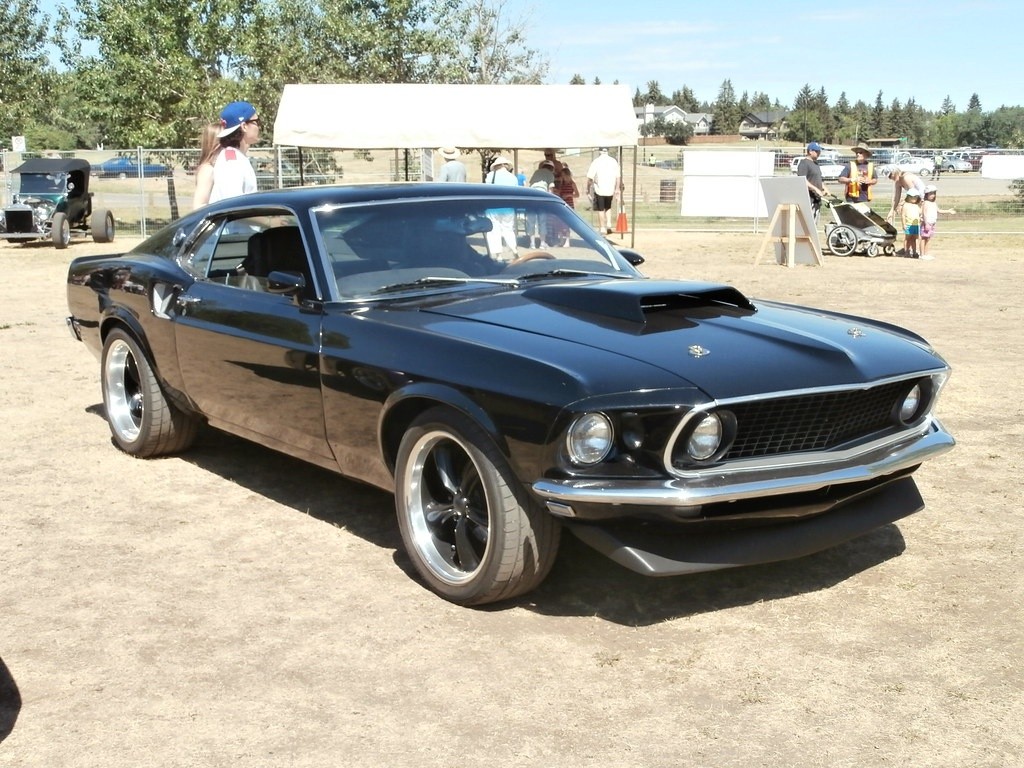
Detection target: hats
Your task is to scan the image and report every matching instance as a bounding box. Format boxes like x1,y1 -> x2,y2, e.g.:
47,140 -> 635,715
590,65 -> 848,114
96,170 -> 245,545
599,147 -> 609,152
539,160 -> 555,173
438,144 -> 460,159
490,157 -> 513,172
851,143 -> 872,158
906,188 -> 920,197
216,101 -> 258,138
882,167 -> 895,178
923,185 -> 938,193
807,142 -> 825,151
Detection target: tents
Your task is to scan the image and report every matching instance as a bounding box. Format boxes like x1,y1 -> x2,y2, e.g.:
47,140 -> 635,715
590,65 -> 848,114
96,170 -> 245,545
270,84 -> 639,249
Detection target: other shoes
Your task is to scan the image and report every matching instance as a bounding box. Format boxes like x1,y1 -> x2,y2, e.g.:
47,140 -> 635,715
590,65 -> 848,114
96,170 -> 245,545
905,253 -> 919,258
607,229 -> 611,234
526,239 -> 571,249
918,254 -> 935,260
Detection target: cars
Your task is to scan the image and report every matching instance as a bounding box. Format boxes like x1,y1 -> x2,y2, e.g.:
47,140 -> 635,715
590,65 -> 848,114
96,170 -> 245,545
0,158 -> 115,248
790,156 -> 803,172
90,157 -> 170,179
66,182 -> 955,607
918,152 -> 988,172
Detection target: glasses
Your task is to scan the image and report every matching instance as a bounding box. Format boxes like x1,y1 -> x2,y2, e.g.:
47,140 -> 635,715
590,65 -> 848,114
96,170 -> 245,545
246,118 -> 260,126
856,152 -> 863,154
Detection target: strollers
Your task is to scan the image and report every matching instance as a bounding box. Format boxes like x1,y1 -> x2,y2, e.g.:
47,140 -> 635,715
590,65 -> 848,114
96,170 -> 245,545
815,191 -> 898,257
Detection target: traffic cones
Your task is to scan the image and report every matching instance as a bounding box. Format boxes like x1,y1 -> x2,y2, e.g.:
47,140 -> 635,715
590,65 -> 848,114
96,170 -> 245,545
613,201 -> 631,234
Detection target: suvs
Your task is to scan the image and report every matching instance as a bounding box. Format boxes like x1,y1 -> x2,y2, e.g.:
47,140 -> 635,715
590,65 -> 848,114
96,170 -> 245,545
817,158 -> 846,180
884,157 -> 935,177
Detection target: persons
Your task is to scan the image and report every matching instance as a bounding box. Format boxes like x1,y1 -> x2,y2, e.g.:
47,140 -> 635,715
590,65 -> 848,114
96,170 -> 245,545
648,154 -> 656,167
482,156 -> 580,263
838,142 -> 878,204
586,147 -> 622,235
193,102 -> 261,210
881,165 -> 956,260
438,144 -> 466,183
797,142 -> 831,228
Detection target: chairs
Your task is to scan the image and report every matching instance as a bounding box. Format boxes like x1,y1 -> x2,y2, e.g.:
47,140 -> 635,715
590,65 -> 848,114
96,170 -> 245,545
246,225 -> 316,300
